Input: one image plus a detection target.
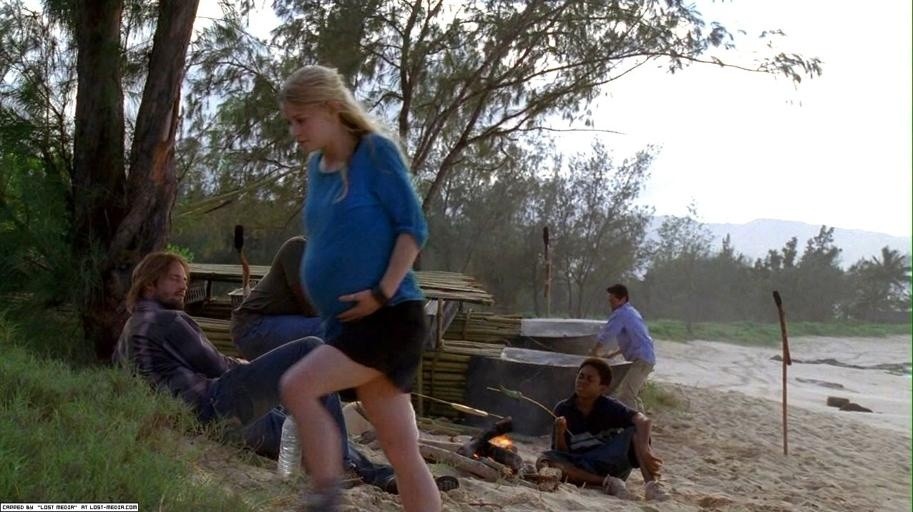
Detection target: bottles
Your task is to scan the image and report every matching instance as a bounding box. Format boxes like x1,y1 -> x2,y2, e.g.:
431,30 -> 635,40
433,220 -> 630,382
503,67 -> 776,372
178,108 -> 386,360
276,410 -> 305,479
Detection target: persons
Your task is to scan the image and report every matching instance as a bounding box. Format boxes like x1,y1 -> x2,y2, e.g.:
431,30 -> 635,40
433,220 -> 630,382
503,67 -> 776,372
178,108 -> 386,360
582,284 -> 655,416
535,357 -> 670,501
273,61 -> 443,512
109,252 -> 460,498
226,234 -> 363,488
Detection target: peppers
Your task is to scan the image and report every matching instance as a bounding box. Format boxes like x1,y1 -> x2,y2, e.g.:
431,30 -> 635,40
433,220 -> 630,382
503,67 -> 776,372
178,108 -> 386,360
499,385 -> 521,401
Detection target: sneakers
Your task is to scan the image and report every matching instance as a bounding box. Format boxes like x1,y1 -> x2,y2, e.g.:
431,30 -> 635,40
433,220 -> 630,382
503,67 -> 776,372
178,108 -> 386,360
381,472 -> 461,494
644,477 -> 670,502
600,475 -> 632,499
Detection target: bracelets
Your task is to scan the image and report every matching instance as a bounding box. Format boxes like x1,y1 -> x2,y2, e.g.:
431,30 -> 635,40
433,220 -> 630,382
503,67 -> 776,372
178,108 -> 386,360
370,283 -> 389,306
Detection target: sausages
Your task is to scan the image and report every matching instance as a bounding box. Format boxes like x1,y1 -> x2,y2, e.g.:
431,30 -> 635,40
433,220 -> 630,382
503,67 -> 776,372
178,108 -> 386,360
452,403 -> 489,418
